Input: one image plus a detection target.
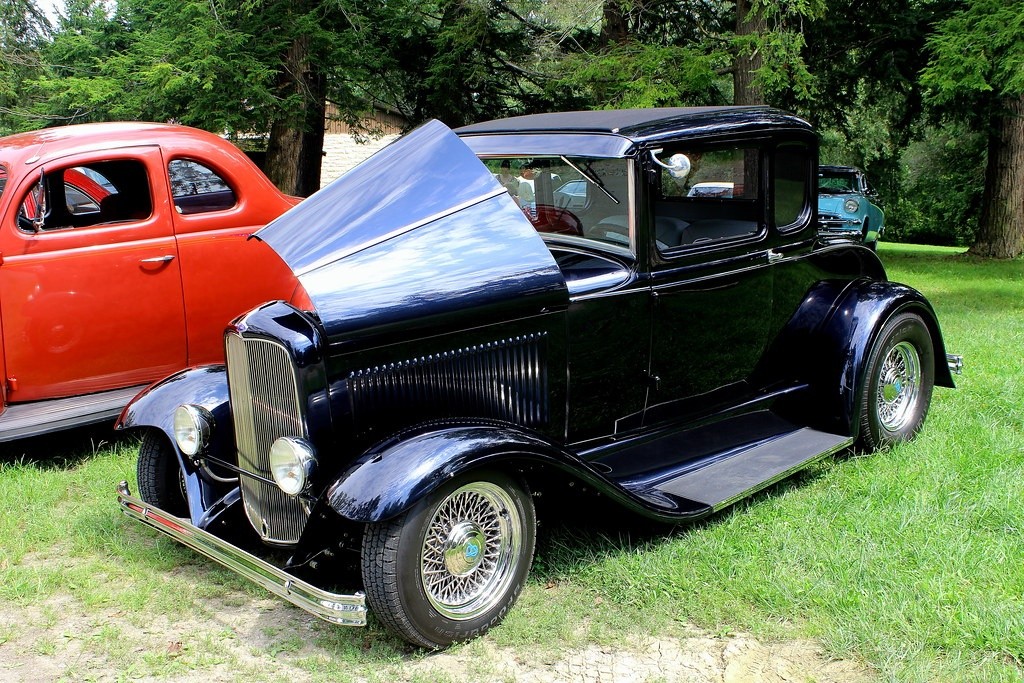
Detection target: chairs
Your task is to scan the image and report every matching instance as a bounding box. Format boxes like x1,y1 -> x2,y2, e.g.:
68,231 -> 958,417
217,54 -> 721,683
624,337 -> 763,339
682,220 -> 758,244
597,215 -> 688,249
100,193 -> 147,220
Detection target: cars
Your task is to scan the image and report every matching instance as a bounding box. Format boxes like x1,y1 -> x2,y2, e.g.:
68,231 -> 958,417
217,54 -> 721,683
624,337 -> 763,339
685,182 -> 739,202
813,163 -> 888,252
0,119 -> 311,467
527,177 -> 591,213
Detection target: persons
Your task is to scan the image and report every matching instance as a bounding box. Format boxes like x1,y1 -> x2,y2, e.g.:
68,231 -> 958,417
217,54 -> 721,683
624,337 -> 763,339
495,159 -> 520,194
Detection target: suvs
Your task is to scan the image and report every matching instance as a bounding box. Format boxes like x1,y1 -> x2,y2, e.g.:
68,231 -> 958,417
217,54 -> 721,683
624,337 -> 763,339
112,103 -> 965,650
